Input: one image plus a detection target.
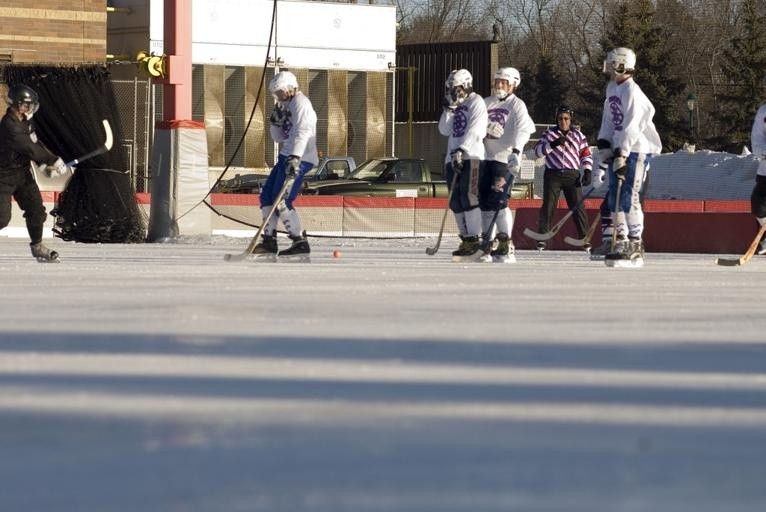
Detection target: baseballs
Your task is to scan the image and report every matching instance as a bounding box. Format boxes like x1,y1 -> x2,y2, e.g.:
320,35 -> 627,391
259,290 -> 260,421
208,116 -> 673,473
333,250 -> 342,257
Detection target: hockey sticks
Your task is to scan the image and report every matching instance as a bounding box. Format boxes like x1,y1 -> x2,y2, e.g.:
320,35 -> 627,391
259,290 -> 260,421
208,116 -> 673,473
522,173 -> 604,241
424,173 -> 458,255
65,120 -> 113,169
715,222 -> 766,267
588,179 -> 621,261
565,191 -> 605,247
224,176 -> 290,263
452,176 -> 512,263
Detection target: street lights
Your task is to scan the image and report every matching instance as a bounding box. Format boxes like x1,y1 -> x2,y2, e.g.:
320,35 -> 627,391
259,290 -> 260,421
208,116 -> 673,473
686,90 -> 696,146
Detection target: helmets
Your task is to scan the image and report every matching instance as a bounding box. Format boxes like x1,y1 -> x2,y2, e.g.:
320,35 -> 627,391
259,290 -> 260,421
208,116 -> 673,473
554,102 -> 574,125
268,70 -> 298,101
491,67 -> 522,100
6,83 -> 40,120
443,69 -> 474,105
602,47 -> 638,81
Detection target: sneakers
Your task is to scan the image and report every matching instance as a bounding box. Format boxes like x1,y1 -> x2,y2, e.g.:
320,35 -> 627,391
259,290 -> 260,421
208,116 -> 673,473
581,237 -> 644,260
29,242 -> 59,261
251,229 -> 279,254
757,235 -> 766,255
536,241 -> 546,250
451,233 -> 516,257
278,230 -> 311,255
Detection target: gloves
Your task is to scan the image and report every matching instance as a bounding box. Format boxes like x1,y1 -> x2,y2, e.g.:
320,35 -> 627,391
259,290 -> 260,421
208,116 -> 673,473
449,147 -> 465,173
442,87 -> 465,112
612,153 -> 628,182
580,168 -> 590,189
269,103 -> 289,127
549,135 -> 566,148
283,154 -> 301,180
44,156 -> 69,179
507,152 -> 521,179
593,160 -> 610,191
487,121 -> 505,139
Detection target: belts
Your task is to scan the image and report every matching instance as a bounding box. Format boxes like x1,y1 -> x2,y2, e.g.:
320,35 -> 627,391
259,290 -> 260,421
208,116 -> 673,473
546,169 -> 578,173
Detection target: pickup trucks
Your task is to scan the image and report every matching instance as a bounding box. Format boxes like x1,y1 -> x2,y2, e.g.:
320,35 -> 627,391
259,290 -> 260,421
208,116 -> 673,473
300,154 -> 533,200
208,150 -> 358,194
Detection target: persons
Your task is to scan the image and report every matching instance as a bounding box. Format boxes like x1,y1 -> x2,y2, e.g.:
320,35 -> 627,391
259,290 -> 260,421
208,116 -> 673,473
751,103 -> 766,255
478,66 -> 537,257
252,70 -> 318,256
589,47 -> 663,261
533,105 -> 593,249
0,85 -> 67,260
436,68 -> 488,256
